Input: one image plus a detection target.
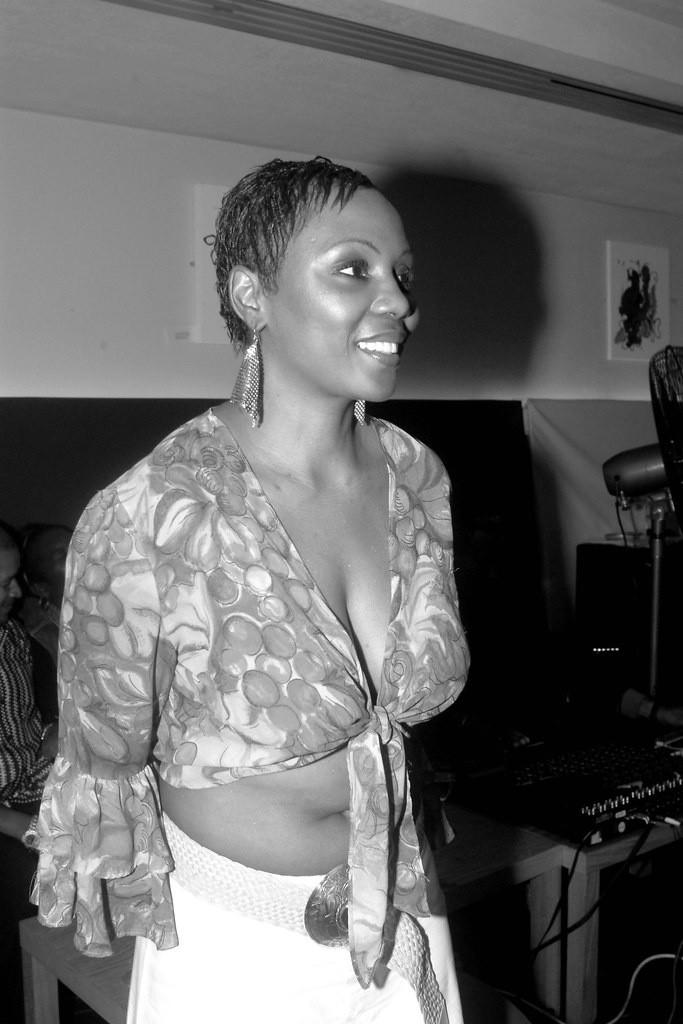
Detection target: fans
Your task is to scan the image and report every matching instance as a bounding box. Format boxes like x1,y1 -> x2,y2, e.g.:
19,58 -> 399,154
602,345 -> 682,703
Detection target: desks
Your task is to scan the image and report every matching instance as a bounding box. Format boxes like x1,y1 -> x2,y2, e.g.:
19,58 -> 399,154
1,794 -> 683,1023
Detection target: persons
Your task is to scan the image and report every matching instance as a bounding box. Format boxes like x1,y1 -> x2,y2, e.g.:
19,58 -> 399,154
0,522 -> 73,1024
21,155 -> 470,1024
